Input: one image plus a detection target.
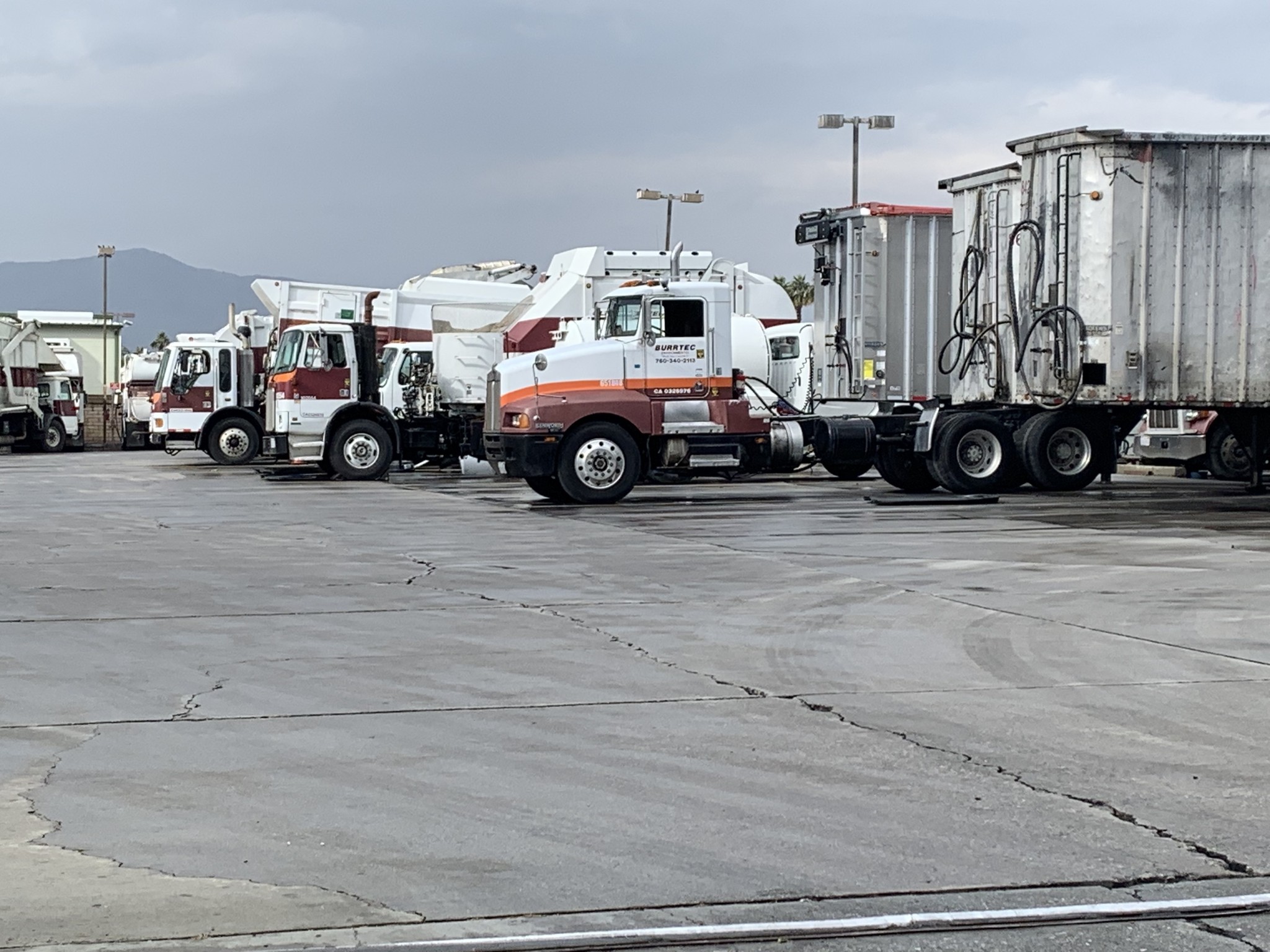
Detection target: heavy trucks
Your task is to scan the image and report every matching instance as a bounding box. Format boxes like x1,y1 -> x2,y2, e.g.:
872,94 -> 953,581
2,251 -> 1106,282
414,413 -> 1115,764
112,201 -> 987,482
0,312 -> 88,455
475,124 -> 1270,507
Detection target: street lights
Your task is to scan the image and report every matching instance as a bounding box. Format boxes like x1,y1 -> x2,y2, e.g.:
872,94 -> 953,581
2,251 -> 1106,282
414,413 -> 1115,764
816,114 -> 895,207
636,188 -> 705,252
